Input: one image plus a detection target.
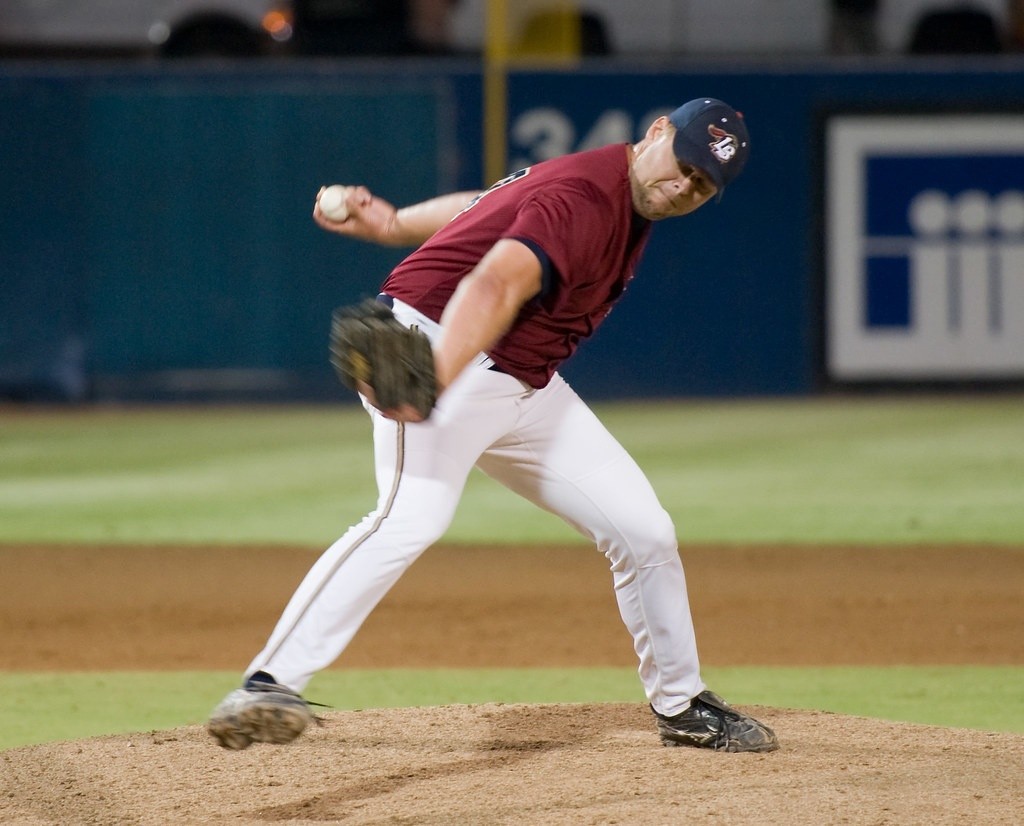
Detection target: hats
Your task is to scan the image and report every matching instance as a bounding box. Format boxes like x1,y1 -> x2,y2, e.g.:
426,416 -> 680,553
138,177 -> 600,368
669,97 -> 751,205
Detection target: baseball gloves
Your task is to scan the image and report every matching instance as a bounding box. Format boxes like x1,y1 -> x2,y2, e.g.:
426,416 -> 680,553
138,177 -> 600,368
324,297 -> 439,422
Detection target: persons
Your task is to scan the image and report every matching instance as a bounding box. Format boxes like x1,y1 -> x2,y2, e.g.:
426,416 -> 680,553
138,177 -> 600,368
829,0 -> 1024,56
204,97 -> 777,752
292,0 -> 455,57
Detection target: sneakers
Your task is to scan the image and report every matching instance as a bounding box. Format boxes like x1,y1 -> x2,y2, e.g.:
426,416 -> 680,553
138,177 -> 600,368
208,670 -> 312,751
649,689 -> 779,753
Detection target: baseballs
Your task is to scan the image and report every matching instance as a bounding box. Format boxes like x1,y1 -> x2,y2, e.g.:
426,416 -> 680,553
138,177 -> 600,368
318,184 -> 352,223
